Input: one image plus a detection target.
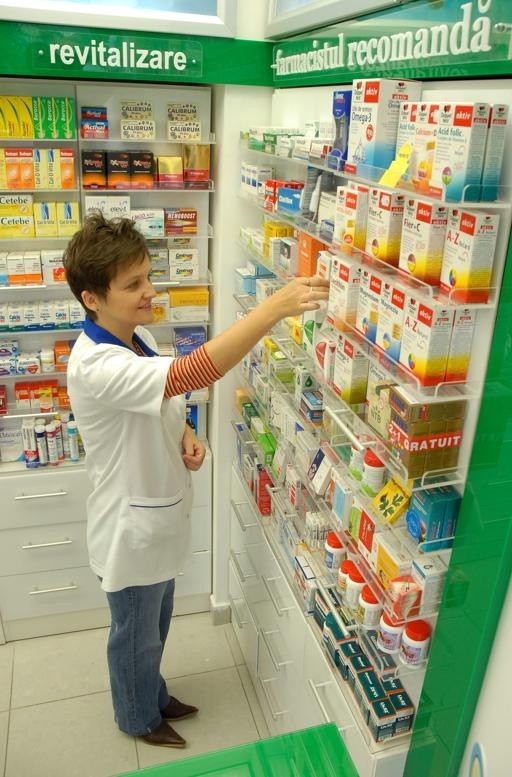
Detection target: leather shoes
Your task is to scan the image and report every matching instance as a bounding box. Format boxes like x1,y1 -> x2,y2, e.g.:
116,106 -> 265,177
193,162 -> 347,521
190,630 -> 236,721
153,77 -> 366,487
145,695 -> 199,750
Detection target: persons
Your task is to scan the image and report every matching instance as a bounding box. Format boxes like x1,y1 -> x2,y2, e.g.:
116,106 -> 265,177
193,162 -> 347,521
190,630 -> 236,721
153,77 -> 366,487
62,208 -> 330,749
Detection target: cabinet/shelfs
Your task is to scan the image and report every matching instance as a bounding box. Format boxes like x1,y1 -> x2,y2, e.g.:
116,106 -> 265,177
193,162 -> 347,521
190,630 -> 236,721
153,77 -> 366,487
1,0 -> 258,648
233,137 -> 509,772
